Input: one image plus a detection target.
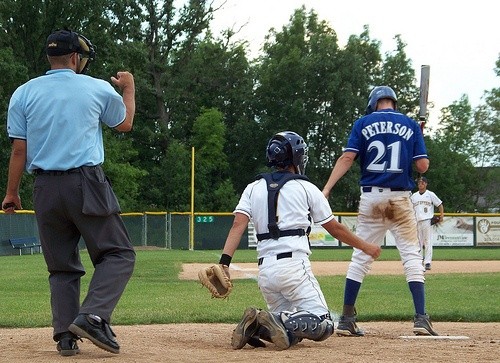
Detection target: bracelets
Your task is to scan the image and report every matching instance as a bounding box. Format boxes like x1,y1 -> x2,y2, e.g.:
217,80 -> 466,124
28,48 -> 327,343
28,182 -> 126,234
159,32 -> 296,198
219,253 -> 232,267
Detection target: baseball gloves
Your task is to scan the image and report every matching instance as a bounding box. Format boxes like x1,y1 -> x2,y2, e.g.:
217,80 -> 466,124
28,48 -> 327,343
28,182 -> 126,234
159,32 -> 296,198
431,216 -> 441,225
198,264 -> 233,299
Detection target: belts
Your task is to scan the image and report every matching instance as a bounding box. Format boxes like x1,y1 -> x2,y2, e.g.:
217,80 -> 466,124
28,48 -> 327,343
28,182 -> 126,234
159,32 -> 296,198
35,168 -> 72,176
363,186 -> 406,192
258,251 -> 292,265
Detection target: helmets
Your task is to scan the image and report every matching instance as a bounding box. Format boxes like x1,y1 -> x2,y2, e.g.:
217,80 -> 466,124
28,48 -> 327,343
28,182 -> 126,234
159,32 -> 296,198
366,86 -> 398,115
265,131 -> 309,176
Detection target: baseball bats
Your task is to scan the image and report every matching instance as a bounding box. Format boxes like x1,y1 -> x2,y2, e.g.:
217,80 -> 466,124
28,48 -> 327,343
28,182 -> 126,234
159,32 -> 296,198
420,65 -> 430,132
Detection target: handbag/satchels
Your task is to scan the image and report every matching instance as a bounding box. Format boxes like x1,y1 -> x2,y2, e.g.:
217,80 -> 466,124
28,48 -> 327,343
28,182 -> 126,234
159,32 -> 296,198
78,165 -> 122,216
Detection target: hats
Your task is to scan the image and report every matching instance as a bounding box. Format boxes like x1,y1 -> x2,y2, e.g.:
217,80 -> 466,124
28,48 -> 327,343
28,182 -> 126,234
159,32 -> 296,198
46,32 -> 75,56
415,176 -> 427,183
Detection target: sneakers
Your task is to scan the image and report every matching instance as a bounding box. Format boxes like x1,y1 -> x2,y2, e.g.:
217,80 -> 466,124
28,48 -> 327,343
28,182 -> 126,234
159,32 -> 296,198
56,334 -> 83,356
336,314 -> 365,336
257,310 -> 290,350
425,263 -> 432,270
412,312 -> 438,336
67,314 -> 119,354
231,307 -> 259,351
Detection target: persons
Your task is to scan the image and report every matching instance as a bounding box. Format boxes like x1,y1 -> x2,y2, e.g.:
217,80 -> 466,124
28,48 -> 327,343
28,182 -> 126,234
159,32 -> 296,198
323,86 -> 440,337
199,131 -> 381,350
2,30 -> 137,356
411,176 -> 444,271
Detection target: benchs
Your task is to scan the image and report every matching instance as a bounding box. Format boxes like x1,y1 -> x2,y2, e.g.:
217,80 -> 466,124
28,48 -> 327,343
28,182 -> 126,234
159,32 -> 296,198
9,237 -> 41,255
